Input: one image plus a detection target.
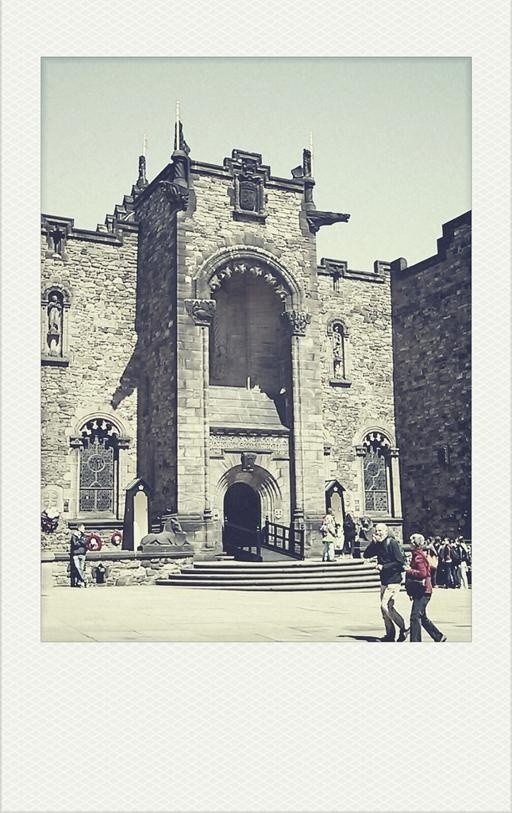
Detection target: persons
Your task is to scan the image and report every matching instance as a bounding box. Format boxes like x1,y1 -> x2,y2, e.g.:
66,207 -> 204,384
364,523 -> 411,641
321,513 -> 338,560
69,523 -> 88,588
422,534 -> 471,589
321,507 -> 339,560
343,513 -> 357,555
402,532 -> 448,641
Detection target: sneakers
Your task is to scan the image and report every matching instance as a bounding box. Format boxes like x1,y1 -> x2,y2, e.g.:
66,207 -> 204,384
380,635 -> 395,642
397,627 -> 410,642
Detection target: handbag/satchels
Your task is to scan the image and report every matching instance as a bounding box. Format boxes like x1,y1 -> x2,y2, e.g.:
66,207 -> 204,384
406,578 -> 425,599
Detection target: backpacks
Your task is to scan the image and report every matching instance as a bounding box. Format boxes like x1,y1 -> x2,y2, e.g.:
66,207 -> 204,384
395,540 -> 406,561
